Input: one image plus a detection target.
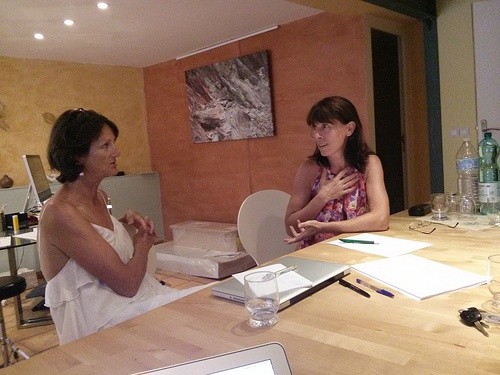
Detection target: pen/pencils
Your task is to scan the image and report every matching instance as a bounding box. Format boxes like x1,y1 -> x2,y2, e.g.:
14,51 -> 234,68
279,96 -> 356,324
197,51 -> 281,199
356,279 -> 395,297
339,279 -> 371,298
263,265 -> 298,279
339,238 -> 377,244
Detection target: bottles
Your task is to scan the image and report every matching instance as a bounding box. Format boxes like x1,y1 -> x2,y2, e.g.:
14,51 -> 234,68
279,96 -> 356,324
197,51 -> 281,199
477,132 -> 500,215
455,137 -> 479,211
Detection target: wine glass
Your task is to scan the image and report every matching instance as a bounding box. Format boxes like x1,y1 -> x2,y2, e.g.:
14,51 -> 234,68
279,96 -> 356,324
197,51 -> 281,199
485,254 -> 500,323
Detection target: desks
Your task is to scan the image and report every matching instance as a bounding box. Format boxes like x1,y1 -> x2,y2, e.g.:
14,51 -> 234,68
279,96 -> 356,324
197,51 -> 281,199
0,223 -> 54,329
0,210 -> 500,375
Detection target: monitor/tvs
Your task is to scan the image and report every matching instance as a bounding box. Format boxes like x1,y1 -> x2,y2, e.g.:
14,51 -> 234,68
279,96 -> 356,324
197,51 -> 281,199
23,155 -> 51,206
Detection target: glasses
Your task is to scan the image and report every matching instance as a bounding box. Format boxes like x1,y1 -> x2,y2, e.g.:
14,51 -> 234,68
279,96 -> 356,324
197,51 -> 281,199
409,219 -> 459,234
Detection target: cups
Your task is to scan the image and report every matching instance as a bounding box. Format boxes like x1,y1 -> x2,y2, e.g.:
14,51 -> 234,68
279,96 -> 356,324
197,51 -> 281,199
244,271 -> 279,329
429,191 -> 500,227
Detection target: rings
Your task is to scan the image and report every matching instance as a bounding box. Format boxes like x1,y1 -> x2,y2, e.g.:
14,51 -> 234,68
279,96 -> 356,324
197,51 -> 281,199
145,216 -> 149,220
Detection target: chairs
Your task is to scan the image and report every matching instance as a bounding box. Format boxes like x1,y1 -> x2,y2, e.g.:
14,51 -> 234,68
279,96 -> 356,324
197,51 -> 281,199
237,189 -> 301,266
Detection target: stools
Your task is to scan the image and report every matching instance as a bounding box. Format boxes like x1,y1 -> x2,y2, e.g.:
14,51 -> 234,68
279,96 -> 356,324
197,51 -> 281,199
0,276 -> 27,369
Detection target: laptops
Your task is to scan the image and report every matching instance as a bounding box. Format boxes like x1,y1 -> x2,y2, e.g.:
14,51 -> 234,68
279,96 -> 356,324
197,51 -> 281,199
133,342 -> 292,375
211,255 -> 350,311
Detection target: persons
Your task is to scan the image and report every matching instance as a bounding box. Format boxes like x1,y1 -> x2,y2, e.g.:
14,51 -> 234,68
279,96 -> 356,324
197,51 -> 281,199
36,108 -> 220,346
283,96 -> 390,253
